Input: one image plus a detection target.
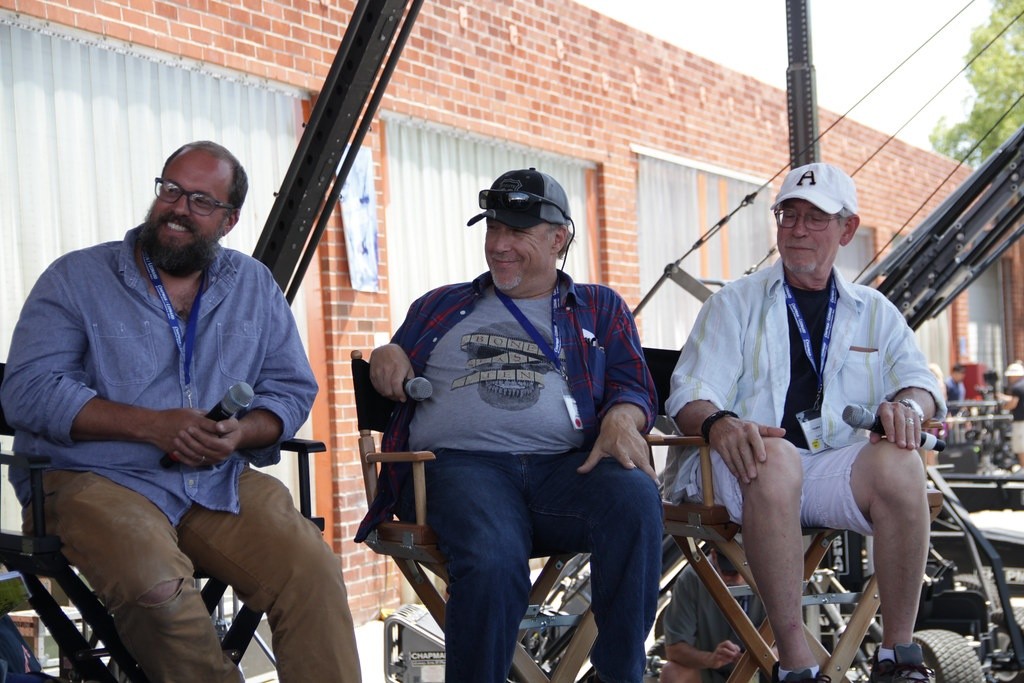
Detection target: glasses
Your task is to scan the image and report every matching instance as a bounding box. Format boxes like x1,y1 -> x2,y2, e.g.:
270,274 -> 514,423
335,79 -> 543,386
774,206 -> 838,232
479,189 -> 564,215
154,177 -> 234,216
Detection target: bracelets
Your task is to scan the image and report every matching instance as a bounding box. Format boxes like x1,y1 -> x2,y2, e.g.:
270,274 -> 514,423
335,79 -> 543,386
701,410 -> 738,444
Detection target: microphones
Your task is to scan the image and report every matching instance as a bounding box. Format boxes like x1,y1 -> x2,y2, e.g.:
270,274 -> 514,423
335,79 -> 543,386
403,377 -> 433,401
160,382 -> 254,468
843,405 -> 947,453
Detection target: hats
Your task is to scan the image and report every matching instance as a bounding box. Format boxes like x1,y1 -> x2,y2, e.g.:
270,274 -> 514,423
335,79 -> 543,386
466,167 -> 571,229
770,162 -> 857,216
717,533 -> 743,571
1003,363 -> 1024,377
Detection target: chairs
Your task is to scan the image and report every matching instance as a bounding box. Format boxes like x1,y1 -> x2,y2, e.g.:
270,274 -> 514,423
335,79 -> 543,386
0,357 -> 330,683
637,342 -> 948,683
349,345 -> 687,683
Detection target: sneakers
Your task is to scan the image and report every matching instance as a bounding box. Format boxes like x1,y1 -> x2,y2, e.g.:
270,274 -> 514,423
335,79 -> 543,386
869,643 -> 935,683
771,661 -> 833,683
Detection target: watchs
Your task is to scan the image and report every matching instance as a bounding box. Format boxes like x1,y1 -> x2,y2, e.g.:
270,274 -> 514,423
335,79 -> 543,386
897,399 -> 924,421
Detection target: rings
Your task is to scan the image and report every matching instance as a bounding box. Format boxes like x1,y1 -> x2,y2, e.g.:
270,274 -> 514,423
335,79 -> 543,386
906,419 -> 914,424
200,456 -> 205,463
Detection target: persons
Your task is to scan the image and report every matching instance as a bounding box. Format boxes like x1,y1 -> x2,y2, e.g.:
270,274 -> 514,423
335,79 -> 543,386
994,363 -> 1024,465
662,162 -> 947,683
1,141 -> 363,683
944,366 -> 973,445
353,168 -> 663,683
659,533 -> 780,683
